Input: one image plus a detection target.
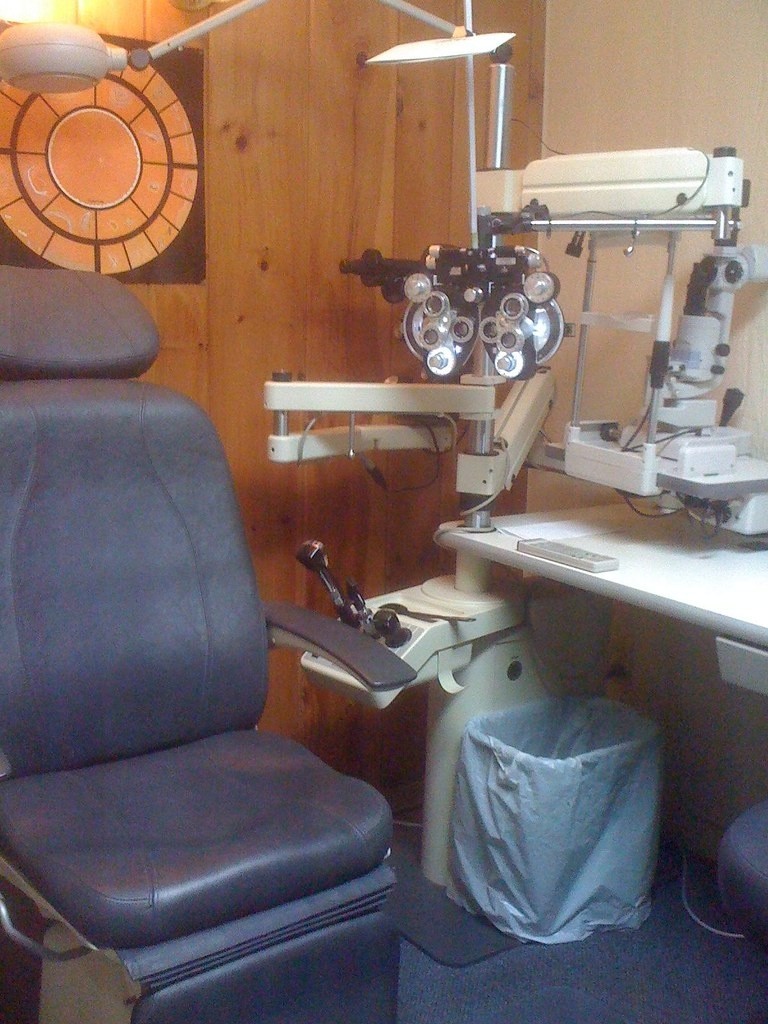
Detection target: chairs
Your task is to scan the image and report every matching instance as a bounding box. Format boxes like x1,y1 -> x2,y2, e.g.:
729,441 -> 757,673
0,268 -> 419,1024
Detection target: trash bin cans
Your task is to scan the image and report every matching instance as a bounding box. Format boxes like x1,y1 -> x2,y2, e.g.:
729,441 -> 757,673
454,695 -> 661,933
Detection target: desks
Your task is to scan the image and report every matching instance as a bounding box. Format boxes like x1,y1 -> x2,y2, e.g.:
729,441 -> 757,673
434,504 -> 768,695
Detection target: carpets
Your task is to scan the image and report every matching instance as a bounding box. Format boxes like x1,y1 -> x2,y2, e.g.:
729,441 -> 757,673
397,845 -> 768,1024
